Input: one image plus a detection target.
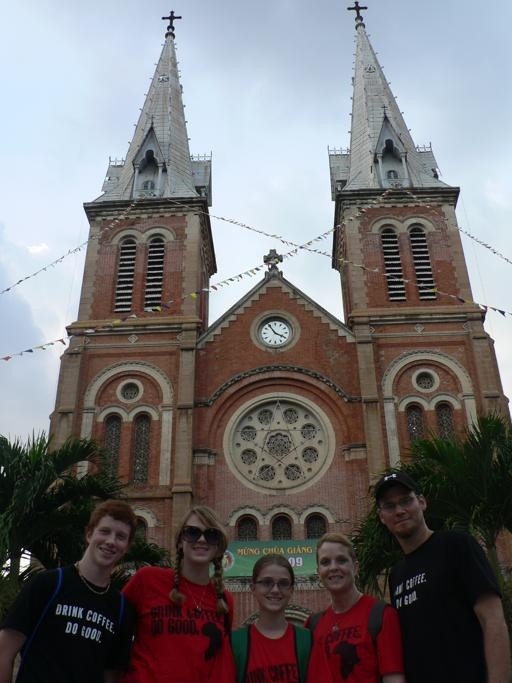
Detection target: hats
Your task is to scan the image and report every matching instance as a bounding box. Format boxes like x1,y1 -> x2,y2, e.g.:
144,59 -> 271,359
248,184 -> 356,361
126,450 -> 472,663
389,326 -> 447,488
374,471 -> 420,507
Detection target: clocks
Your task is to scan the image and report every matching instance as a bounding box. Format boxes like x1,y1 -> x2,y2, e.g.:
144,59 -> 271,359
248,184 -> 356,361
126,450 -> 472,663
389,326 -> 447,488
257,314 -> 294,348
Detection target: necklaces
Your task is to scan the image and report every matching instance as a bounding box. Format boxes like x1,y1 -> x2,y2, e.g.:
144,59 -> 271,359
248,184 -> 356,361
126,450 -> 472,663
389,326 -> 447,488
180,574 -> 209,617
330,591 -> 359,635
75,560 -> 112,596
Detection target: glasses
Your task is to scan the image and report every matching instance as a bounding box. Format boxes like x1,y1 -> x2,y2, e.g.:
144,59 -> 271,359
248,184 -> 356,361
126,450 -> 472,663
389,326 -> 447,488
181,525 -> 221,546
255,578 -> 294,588
377,494 -> 418,510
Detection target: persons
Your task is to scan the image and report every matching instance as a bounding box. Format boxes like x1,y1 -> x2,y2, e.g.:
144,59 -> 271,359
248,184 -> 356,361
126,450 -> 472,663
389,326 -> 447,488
121,506 -> 236,683
374,473 -> 512,683
303,532 -> 406,683
0,499 -> 140,683
230,553 -> 316,683
